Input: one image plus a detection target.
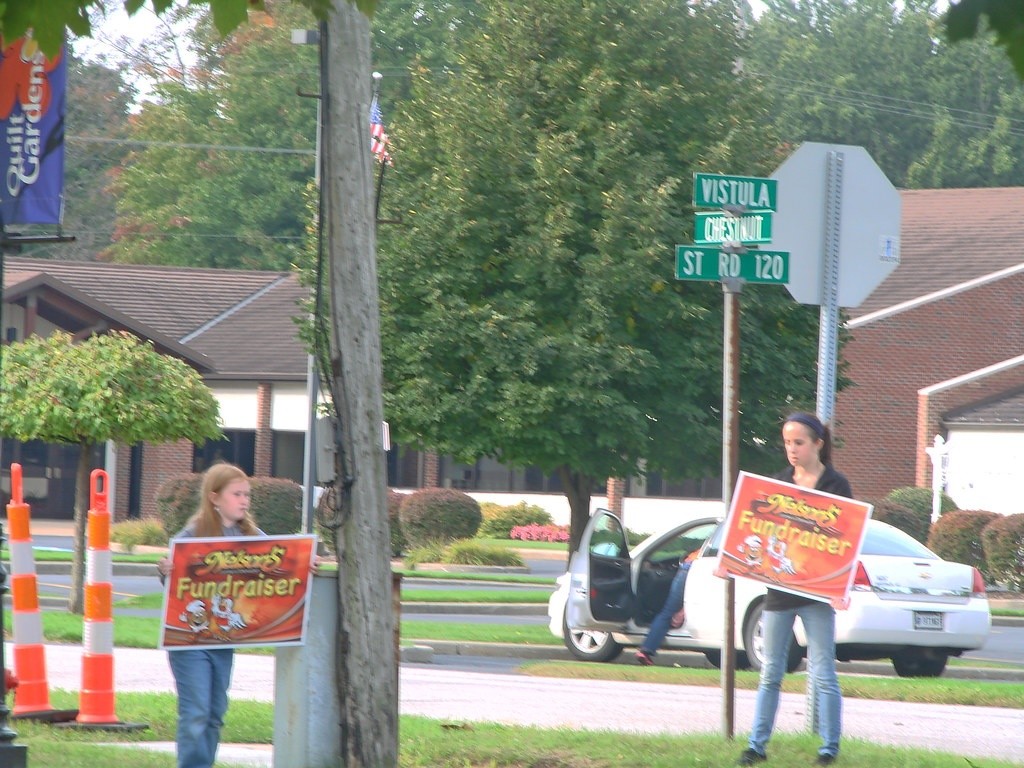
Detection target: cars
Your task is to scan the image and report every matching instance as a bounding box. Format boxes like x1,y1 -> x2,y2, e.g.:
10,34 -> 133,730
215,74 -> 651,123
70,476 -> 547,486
548,506 -> 992,680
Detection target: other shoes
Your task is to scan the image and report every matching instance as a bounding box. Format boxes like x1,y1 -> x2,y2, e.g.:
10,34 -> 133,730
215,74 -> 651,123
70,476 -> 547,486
814,751 -> 833,767
635,651 -> 655,667
670,610 -> 684,629
739,750 -> 767,767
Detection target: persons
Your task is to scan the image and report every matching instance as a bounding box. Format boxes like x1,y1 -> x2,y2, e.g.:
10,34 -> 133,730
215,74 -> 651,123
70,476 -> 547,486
635,549 -> 701,665
734,412 -> 854,767
156,464 -> 322,768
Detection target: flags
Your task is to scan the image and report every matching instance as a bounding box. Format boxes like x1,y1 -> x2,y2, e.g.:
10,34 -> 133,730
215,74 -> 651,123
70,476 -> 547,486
370,103 -> 392,166
